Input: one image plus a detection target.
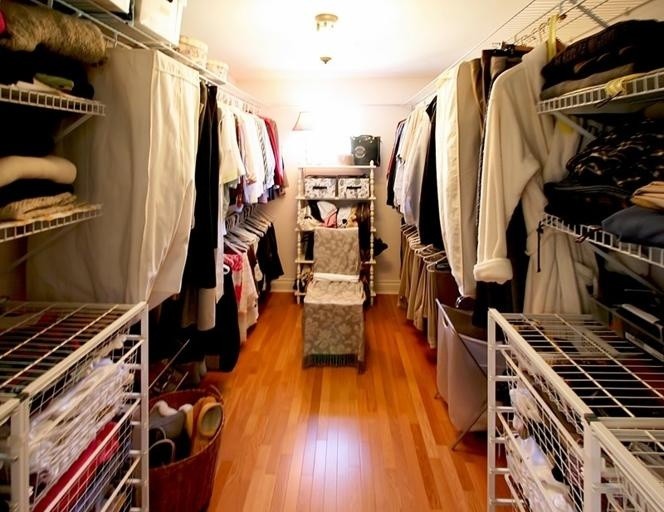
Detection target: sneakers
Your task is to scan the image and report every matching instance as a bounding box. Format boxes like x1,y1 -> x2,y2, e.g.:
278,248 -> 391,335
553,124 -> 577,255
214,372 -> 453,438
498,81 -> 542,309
149,358 -> 223,470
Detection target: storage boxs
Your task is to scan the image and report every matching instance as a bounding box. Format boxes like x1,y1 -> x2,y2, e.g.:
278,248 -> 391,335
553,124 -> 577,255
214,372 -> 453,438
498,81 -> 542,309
104,0 -> 184,46
208,61 -> 229,81
183,37 -> 208,68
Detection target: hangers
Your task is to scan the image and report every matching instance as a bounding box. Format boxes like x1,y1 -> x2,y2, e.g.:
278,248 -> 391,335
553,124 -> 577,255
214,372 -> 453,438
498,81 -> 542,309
426,255 -> 451,273
508,15 -> 553,48
215,90 -> 272,119
222,204 -> 276,275
423,250 -> 446,264
393,220 -> 438,257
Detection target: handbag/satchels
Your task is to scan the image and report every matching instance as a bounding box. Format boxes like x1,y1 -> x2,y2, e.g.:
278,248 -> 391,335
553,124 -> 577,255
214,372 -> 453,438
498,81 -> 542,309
350,135 -> 380,165
544,121 -> 663,227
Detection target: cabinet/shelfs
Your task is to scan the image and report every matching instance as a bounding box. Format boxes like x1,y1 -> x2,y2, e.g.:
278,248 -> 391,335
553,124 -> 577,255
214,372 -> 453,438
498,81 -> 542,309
148,339 -> 200,390
0,295 -> 150,512
295,160 -> 377,306
0,81 -> 105,243
487,306 -> 664,512
536,69 -> 663,267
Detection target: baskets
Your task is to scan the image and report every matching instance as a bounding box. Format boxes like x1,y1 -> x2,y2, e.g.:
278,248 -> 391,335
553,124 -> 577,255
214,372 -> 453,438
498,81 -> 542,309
148,384 -> 223,512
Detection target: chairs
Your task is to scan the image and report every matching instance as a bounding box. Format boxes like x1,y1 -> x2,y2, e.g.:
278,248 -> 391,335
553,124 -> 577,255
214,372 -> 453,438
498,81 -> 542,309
302,228 -> 367,372
